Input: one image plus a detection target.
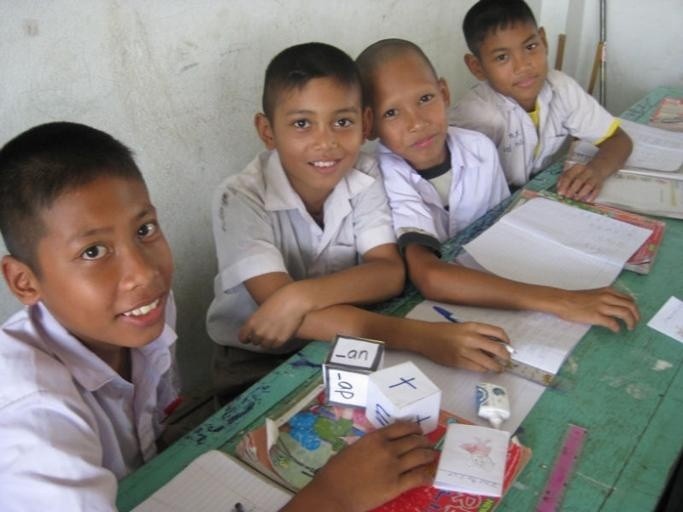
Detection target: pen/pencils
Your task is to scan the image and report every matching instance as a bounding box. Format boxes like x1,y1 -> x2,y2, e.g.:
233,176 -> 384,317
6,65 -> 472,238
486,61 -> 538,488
433,306 -> 516,354
235,502 -> 245,512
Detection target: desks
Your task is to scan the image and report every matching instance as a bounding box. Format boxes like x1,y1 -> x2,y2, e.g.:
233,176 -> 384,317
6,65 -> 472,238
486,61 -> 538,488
112,84 -> 683,512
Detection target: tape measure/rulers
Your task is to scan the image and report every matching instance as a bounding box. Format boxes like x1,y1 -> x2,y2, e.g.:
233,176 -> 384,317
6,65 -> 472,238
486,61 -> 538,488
494,357 -> 576,392
535,423 -> 588,512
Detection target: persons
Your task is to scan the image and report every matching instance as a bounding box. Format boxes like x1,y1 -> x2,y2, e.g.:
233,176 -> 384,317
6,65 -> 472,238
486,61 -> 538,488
447,0 -> 635,204
203,39 -> 515,417
0,120 -> 442,512
355,38 -> 641,334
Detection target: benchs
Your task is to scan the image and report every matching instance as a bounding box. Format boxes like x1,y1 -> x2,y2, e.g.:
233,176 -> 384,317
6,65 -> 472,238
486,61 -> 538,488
166,386 -> 220,430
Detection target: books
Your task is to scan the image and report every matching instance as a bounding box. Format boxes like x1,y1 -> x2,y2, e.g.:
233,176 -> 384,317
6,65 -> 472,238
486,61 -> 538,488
232,374 -> 531,512
381,298 -> 593,436
495,187 -> 666,276
649,97 -> 683,134
565,115 -> 683,223
454,193 -> 653,291
128,448 -> 294,512
